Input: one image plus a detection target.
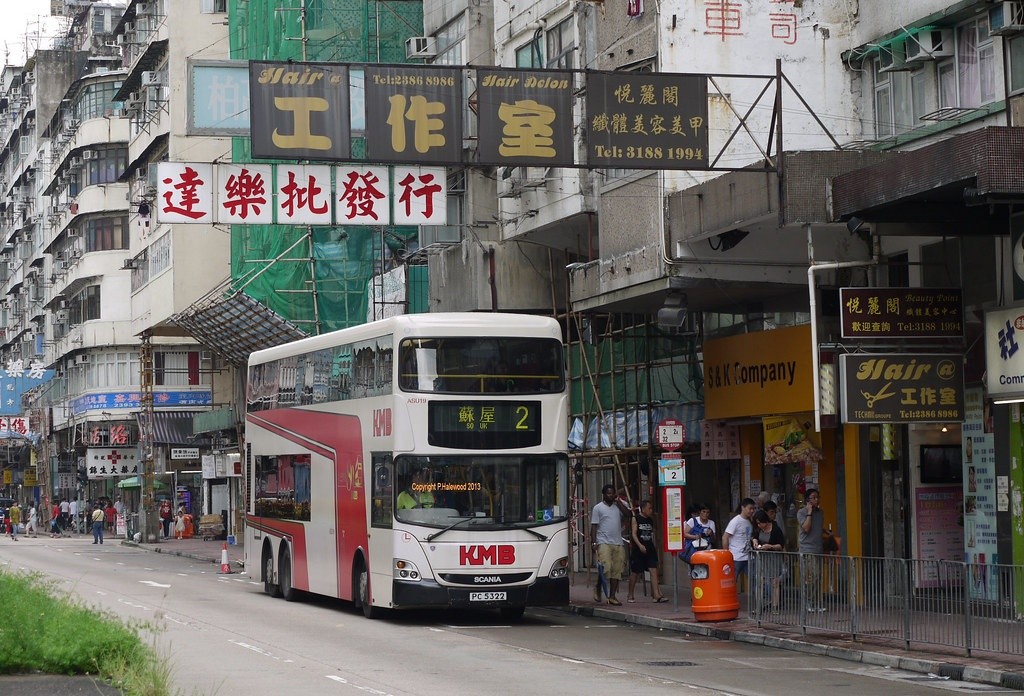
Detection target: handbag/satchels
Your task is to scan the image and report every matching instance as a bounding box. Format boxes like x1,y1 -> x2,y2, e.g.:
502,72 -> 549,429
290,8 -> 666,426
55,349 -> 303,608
18,522 -> 24,528
678,518 -> 712,564
824,536 -> 838,552
91,519 -> 94,524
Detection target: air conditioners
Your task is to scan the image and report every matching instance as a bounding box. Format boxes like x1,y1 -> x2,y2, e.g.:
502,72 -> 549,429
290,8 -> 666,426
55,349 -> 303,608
123,259 -> 137,268
76,354 -> 90,365
141,71 -> 161,87
405,37 -> 436,59
905,29 -> 954,62
119,91 -> 144,119
878,41 -> 922,73
96,66 -> 108,74
136,167 -> 146,181
0,72 -> 84,365
986,0 -> 1024,37
136,2 -> 152,15
83,150 -> 98,160
52,370 -> 62,380
60,365 -> 68,373
117,22 -> 135,46
67,359 -> 78,369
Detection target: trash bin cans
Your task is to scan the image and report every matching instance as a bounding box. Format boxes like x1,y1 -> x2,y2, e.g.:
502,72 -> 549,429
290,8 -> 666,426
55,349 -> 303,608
198,514 -> 223,536
175,514 -> 194,539
690,549 -> 740,621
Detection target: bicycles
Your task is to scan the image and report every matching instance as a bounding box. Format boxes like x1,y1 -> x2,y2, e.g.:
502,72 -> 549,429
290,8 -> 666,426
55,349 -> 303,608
79,518 -> 93,533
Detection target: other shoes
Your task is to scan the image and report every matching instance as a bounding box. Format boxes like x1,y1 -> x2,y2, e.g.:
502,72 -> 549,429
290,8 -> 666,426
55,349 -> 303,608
752,610 -> 755,613
24,535 -> 28,537
50,534 -> 53,537
806,607 -> 815,613
32,535 -> 37,538
817,607 -> 826,612
593,588 -> 601,602
772,610 -> 779,615
92,542 -> 98,544
608,597 -> 623,606
15,539 -> 18,541
57,536 -> 60,538
100,542 -> 103,544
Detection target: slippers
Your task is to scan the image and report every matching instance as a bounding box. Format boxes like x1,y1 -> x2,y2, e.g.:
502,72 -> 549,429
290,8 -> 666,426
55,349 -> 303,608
653,596 -> 669,603
627,597 -> 636,603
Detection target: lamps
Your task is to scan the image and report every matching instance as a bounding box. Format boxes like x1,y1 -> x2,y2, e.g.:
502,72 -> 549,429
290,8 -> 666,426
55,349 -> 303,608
846,215 -> 865,236
581,313 -> 596,346
716,229 -> 750,252
657,291 -> 688,327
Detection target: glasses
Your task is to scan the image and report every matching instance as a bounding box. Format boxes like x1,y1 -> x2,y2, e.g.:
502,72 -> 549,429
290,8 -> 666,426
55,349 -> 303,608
809,495 -> 820,500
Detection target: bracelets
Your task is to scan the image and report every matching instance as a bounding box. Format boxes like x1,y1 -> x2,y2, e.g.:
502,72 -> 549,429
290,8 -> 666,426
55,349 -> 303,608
591,542 -> 596,546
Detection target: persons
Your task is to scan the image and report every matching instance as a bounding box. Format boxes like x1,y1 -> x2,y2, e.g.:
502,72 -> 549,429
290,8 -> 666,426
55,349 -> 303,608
4,501 -> 21,541
722,491 -> 786,615
489,361 -> 518,392
50,502 -> 62,537
494,469 -> 527,523
59,498 -> 70,530
806,515 -> 812,516
160,497 -> 172,539
24,503 -> 38,538
69,495 -> 125,544
685,502 -> 717,602
627,499 -> 669,603
397,462 -> 448,507
173,501 -> 190,540
592,485 -> 638,606
796,489 -> 824,614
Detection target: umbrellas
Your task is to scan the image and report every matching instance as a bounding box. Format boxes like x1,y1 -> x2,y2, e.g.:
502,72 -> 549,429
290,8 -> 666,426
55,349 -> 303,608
116,476 -> 166,489
595,550 -> 609,604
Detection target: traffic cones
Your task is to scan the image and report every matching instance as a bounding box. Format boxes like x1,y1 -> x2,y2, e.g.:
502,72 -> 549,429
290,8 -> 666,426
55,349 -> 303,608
216,542 -> 235,574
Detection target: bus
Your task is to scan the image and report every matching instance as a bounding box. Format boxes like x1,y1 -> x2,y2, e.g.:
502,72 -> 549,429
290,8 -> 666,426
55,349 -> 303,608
244,312 -> 583,619
